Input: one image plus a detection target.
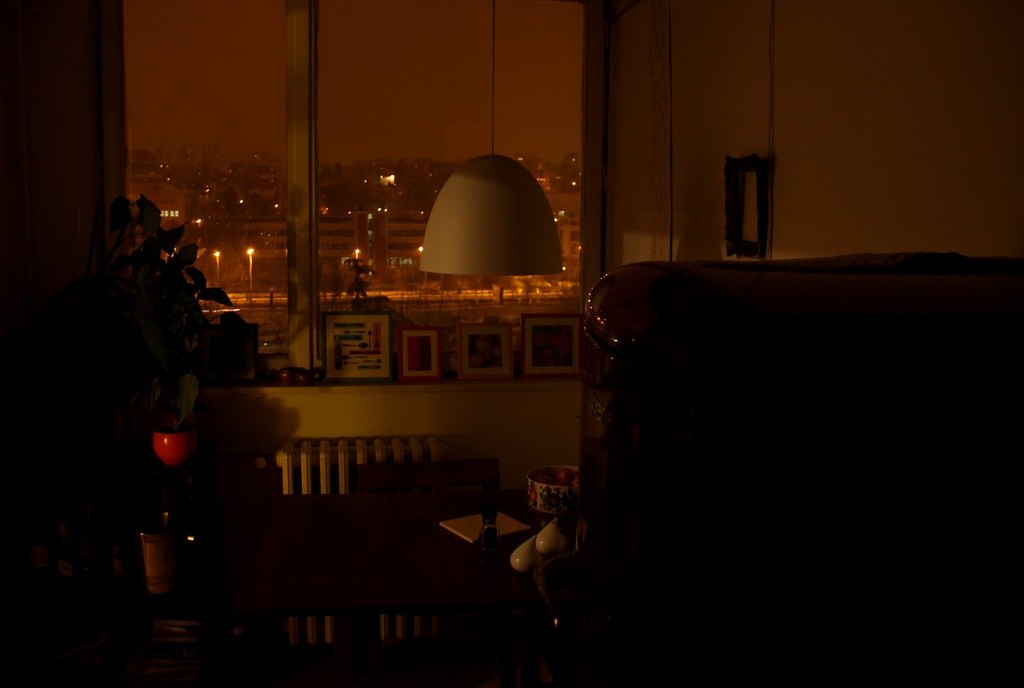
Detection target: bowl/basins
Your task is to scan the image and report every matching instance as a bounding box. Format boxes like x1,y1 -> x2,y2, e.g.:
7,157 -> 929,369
526,464 -> 580,515
152,431 -> 198,466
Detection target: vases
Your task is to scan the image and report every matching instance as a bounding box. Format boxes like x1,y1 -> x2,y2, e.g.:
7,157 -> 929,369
150,425 -> 199,468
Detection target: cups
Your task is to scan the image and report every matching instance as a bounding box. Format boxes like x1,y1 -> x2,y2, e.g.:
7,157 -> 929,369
134,528 -> 176,595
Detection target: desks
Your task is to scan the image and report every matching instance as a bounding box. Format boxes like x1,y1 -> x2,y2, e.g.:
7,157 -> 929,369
233,488 -> 585,688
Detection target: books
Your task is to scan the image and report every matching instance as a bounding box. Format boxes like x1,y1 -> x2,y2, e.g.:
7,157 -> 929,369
439,511 -> 531,543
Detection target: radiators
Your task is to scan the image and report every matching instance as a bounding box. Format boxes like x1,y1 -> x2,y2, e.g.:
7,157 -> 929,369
272,434 -> 453,648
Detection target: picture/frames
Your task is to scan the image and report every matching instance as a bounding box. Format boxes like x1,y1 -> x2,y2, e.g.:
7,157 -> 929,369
398,325 -> 442,383
198,318 -> 260,387
319,310 -> 395,384
456,322 -> 514,381
521,312 -> 586,380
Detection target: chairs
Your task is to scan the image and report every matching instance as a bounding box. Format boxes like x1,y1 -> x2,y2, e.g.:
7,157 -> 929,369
351,453 -> 504,494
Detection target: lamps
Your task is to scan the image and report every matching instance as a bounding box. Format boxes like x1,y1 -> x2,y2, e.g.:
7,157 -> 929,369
420,0 -> 564,276
